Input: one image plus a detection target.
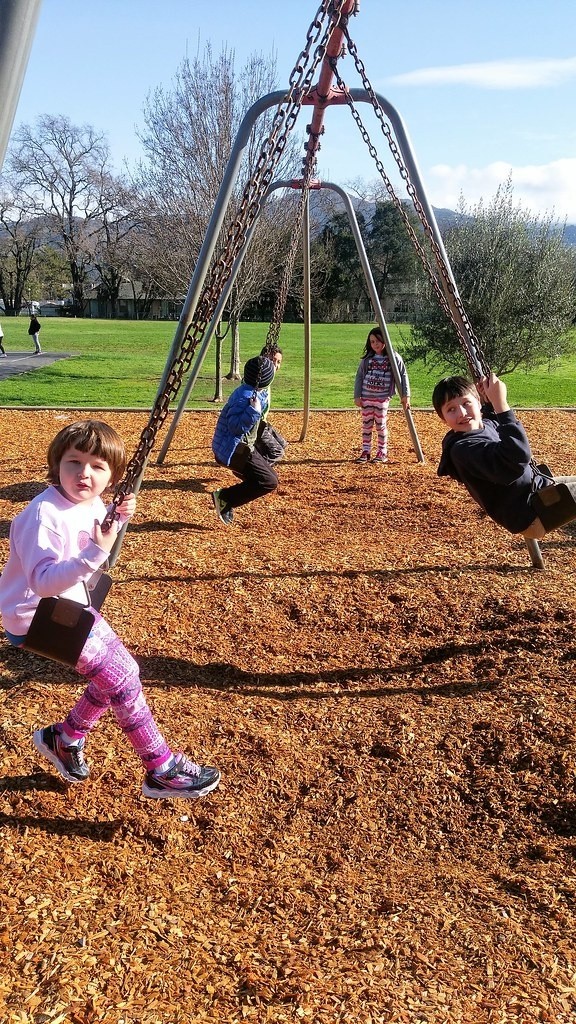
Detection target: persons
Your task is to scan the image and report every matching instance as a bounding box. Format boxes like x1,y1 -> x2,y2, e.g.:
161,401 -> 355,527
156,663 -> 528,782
232,346 -> 287,481
0,328 -> 7,357
353,327 -> 411,463
28,314 -> 41,355
211,355 -> 278,525
0,421 -> 222,798
433,372 -> 576,540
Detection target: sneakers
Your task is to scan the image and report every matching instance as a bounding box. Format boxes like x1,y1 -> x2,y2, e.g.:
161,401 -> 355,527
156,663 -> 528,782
142,754 -> 220,799
211,488 -> 234,524
34,723 -> 90,782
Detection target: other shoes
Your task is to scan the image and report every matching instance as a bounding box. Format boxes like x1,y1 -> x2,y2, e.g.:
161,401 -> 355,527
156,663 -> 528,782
357,453 -> 370,463
33,352 -> 41,354
0,354 -> 7,358
373,455 -> 386,463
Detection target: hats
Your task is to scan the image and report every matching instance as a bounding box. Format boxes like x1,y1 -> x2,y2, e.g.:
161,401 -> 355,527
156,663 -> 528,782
244,356 -> 275,388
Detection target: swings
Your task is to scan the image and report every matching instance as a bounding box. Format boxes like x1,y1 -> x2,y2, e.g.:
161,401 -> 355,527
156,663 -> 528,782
22,1 -> 352,668
323,20 -> 576,539
214,124 -> 326,477
